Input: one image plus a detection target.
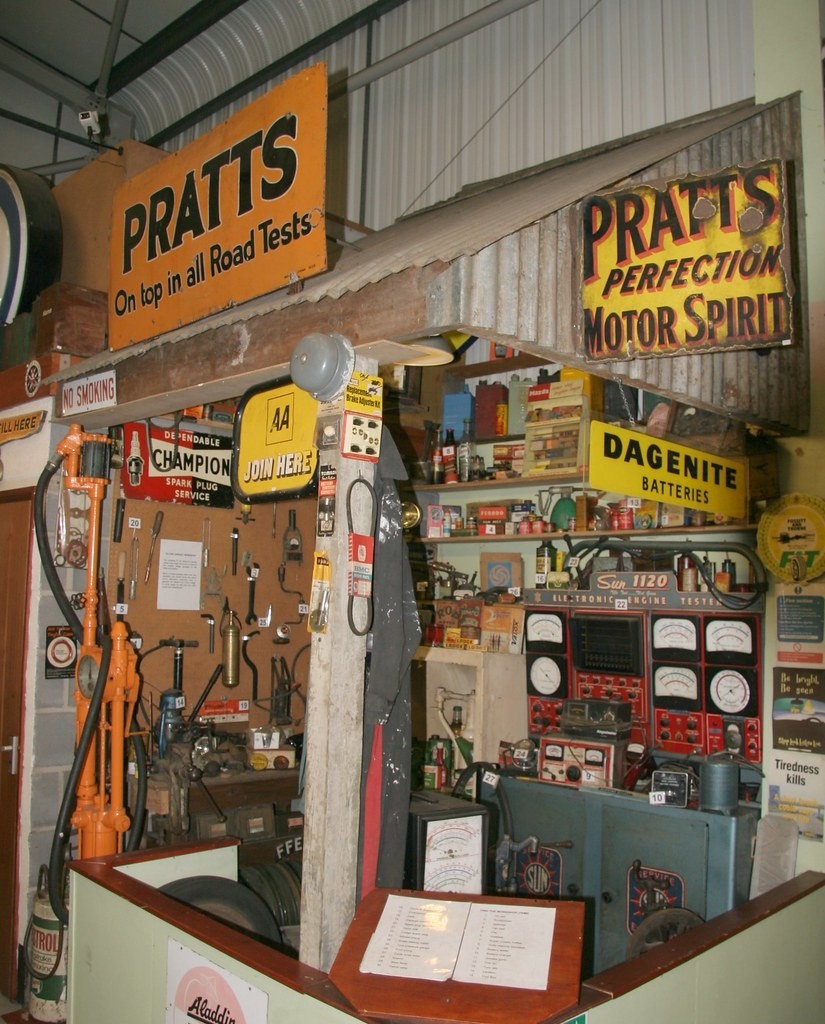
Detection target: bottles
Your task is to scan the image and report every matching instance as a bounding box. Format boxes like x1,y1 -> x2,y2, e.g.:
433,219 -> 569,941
436,742 -> 447,789
701,557 -> 716,593
450,706 -> 462,768
443,507 -> 451,537
442,429 -> 458,484
677,553 -> 698,592
429,735 -> 452,784
459,419 -> 485,482
516,516 -> 533,534
532,515 -> 546,533
524,500 -> 536,514
411,736 -> 431,790
528,507 -> 536,522
723,560 -> 736,591
429,429 -> 445,484
536,540 -> 557,589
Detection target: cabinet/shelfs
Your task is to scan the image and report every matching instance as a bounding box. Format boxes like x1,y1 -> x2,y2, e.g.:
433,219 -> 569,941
401,353 -> 780,543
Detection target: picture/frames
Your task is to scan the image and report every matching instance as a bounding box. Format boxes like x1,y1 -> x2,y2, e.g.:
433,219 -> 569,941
376,363 -> 423,403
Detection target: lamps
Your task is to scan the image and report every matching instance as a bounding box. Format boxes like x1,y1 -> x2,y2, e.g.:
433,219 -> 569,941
403,337 -> 454,366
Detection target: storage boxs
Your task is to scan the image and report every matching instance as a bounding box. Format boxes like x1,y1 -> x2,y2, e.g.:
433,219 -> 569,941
479,552 -> 524,593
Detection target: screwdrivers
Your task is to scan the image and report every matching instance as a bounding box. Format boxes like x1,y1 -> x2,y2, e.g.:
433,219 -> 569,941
144,510 -> 162,582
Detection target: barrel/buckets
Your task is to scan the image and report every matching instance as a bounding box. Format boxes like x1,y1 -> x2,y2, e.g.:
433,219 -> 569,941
443,384 -> 475,440
537,368 -> 560,384
508,374 -> 537,435
560,363 -> 604,412
475,378 -> 509,439
